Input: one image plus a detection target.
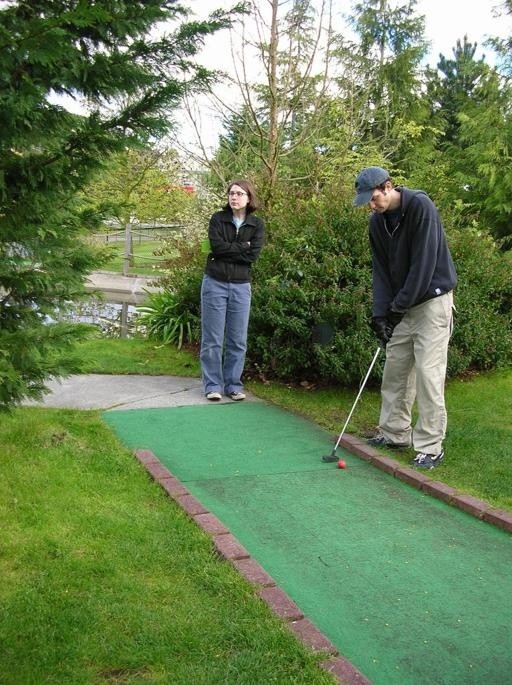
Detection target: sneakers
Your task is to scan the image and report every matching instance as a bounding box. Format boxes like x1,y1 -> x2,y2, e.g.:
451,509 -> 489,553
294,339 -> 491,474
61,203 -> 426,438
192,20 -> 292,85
410,446 -> 445,471
228,391 -> 246,401
206,391 -> 222,401
366,433 -> 411,448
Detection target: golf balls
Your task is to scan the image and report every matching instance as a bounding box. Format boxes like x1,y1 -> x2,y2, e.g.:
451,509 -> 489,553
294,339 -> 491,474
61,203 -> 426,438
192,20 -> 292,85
338,460 -> 346,469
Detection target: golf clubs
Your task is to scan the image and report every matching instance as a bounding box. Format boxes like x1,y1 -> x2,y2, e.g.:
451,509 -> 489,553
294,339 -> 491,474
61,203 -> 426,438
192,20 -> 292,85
323,339 -> 384,462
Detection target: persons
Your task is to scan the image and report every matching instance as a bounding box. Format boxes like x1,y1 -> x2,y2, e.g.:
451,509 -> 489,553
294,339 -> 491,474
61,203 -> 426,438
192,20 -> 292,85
199,180 -> 265,400
351,167 -> 459,470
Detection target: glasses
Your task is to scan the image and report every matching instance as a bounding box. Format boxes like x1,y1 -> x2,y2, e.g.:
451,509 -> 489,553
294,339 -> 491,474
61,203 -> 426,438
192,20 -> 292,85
227,192 -> 248,197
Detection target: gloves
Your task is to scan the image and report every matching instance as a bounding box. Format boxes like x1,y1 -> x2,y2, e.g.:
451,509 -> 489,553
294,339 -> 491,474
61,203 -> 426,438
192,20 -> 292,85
370,307 -> 406,346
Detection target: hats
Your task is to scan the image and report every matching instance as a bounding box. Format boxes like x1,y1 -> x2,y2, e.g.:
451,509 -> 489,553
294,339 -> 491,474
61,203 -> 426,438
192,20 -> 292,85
352,166 -> 390,207
